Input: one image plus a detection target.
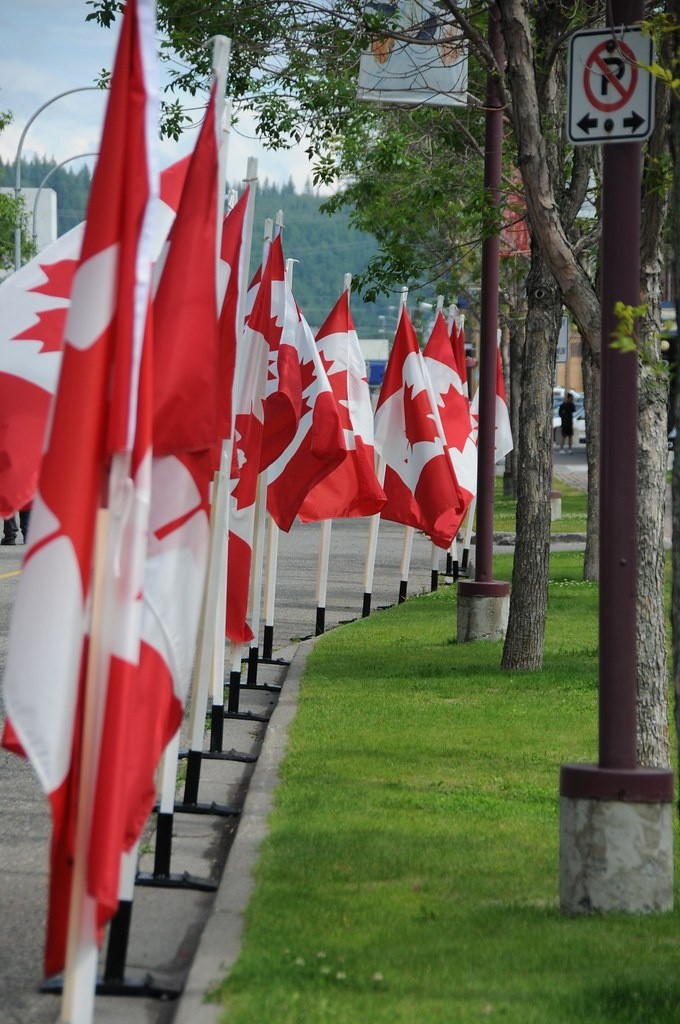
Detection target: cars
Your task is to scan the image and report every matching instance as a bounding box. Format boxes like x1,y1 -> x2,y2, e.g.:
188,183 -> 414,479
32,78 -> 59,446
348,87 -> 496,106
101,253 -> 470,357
549,384 -> 587,447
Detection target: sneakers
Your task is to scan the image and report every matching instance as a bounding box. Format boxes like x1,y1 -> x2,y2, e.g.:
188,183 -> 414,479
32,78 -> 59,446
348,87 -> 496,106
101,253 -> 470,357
1,537 -> 16,545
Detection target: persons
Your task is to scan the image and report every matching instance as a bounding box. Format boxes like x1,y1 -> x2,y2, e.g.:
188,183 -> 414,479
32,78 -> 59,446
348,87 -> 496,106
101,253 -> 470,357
0,509 -> 30,546
559,394 -> 575,454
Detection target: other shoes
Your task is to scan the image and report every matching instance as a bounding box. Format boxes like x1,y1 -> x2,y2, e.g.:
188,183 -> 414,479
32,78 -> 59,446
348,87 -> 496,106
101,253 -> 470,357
559,449 -> 573,454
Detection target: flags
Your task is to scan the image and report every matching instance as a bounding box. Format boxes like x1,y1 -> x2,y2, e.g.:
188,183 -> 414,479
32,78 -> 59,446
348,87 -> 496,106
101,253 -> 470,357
0,0 -> 517,979
